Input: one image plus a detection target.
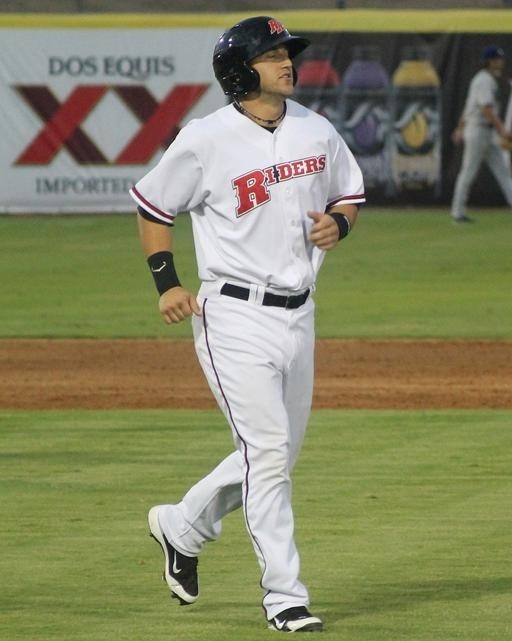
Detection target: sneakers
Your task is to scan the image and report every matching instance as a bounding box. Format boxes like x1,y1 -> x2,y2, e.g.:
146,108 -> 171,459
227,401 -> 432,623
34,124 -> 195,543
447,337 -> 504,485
148,504 -> 199,605
268,606 -> 322,633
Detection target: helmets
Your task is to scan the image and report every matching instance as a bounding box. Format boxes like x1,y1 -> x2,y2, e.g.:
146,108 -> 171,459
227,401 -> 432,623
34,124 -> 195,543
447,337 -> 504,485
213,16 -> 310,96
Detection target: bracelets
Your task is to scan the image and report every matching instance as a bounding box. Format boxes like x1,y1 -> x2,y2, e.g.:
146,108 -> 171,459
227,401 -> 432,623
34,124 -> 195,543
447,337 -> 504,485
329,212 -> 351,242
146,252 -> 181,295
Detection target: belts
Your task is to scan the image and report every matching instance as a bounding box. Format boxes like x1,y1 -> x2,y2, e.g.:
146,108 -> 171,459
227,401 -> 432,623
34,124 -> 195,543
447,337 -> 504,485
221,283 -> 310,309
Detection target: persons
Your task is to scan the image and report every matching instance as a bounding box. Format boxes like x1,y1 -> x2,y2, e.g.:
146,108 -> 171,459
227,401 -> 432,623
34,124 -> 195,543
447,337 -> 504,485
451,48 -> 512,222
129,17 -> 366,632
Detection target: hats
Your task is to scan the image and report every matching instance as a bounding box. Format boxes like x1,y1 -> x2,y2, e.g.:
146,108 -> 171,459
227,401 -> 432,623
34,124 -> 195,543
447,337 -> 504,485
481,46 -> 504,58
